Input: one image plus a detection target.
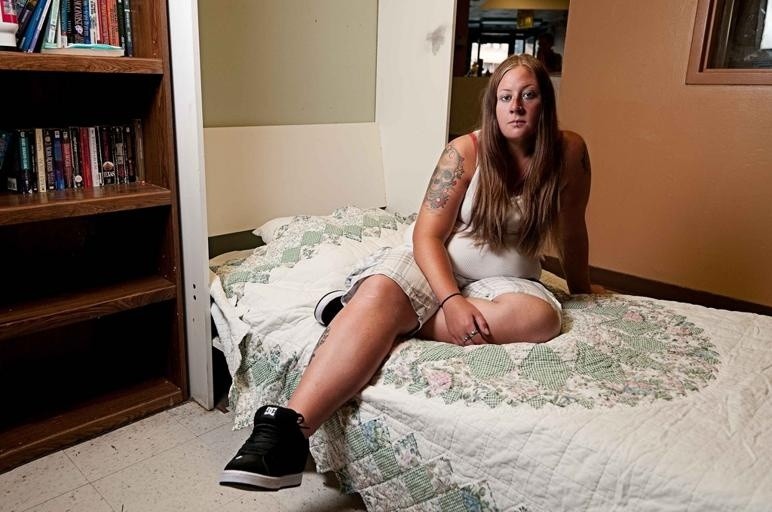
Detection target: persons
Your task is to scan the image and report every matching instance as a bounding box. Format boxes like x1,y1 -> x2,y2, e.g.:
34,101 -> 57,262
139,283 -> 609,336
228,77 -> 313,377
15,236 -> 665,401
217,50 -> 609,494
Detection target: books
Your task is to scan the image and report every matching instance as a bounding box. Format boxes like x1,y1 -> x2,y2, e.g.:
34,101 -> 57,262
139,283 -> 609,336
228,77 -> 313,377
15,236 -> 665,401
0,0 -> 151,195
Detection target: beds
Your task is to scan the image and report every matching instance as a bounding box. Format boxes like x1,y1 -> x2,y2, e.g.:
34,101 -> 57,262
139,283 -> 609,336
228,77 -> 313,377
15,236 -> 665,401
208,208 -> 771,512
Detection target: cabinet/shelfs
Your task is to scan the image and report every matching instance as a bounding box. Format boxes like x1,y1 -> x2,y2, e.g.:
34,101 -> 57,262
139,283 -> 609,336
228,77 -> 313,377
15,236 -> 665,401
0,0 -> 193,474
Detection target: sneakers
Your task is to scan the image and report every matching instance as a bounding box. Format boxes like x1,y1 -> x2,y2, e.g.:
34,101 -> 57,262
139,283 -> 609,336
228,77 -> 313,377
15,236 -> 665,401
314,290 -> 349,326
220,407 -> 308,491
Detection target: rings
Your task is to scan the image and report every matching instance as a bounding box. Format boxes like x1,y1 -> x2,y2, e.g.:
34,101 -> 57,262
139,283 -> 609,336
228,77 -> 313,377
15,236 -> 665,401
469,329 -> 478,339
463,336 -> 471,344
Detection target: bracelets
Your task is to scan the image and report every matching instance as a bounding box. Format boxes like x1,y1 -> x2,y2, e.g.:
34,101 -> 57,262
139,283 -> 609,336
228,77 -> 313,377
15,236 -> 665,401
440,292 -> 463,307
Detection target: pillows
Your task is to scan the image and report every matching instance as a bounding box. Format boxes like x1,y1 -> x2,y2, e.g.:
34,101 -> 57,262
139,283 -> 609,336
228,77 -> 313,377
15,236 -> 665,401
251,204 -> 394,244
